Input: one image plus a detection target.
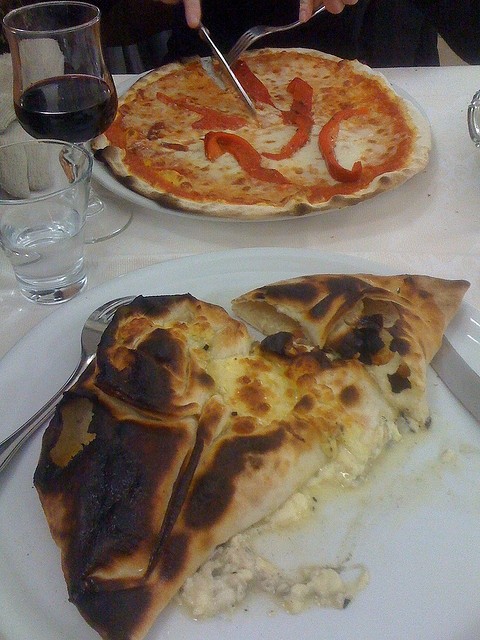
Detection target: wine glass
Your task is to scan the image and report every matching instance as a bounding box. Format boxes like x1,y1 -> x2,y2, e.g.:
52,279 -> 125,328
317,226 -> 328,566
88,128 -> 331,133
3,1 -> 137,246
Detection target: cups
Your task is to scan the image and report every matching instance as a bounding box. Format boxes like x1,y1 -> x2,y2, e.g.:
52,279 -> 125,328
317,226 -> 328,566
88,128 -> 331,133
0,138 -> 94,306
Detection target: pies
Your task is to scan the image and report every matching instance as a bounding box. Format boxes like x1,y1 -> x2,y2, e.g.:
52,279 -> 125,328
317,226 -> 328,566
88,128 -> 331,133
234,272 -> 471,431
33,293 -> 404,640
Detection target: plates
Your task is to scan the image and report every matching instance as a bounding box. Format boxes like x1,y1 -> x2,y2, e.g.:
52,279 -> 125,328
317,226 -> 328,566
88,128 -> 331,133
87,68 -> 434,224
0,247 -> 479,640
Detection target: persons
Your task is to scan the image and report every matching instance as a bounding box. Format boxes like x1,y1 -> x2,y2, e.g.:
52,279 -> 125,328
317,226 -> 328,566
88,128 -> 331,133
100,1 -> 480,76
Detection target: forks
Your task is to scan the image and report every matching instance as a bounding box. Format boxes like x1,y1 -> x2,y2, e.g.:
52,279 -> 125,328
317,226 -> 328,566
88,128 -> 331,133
0,295 -> 150,474
225,3 -> 327,68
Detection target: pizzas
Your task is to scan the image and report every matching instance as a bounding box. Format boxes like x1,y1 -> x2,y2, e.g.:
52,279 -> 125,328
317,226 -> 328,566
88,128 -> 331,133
93,46 -> 435,219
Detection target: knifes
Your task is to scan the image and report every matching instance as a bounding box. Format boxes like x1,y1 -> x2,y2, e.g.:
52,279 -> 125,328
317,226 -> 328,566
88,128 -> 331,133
432,337 -> 478,424
197,20 -> 259,117
